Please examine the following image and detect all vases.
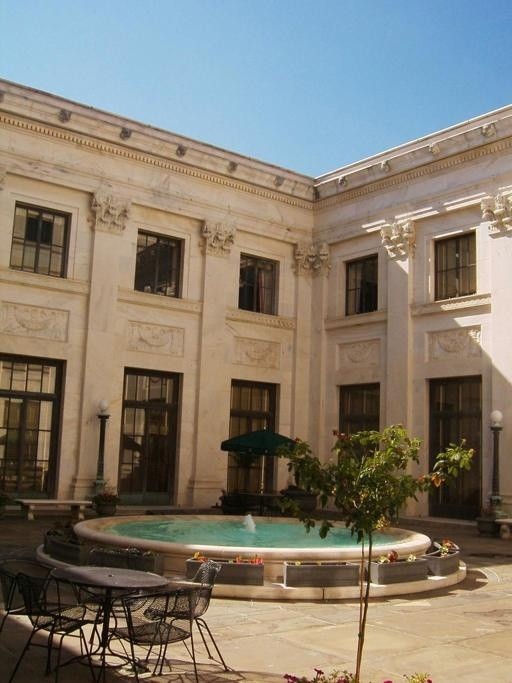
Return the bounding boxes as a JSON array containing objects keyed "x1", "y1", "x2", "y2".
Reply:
[
  {"x1": 280, "y1": 488, "x2": 320, "y2": 514},
  {"x1": 97, "y1": 503, "x2": 116, "y2": 516},
  {"x1": 475, "y1": 518, "x2": 499, "y2": 537}
]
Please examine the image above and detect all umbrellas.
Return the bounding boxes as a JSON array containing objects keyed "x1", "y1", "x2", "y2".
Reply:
[
  {"x1": 0, "y1": 431, "x2": 37, "y2": 459},
  {"x1": 220, "y1": 426, "x2": 296, "y2": 494}
]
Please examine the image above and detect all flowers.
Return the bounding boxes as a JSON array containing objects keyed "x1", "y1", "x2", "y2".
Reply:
[
  {"x1": 90, "y1": 490, "x2": 121, "y2": 504},
  {"x1": 194, "y1": 552, "x2": 208, "y2": 560},
  {"x1": 295, "y1": 560, "x2": 302, "y2": 567},
  {"x1": 378, "y1": 550, "x2": 417, "y2": 563},
  {"x1": 288, "y1": 437, "x2": 321, "y2": 492},
  {"x1": 481, "y1": 505, "x2": 496, "y2": 520},
  {"x1": 233, "y1": 553, "x2": 263, "y2": 564},
  {"x1": 441, "y1": 538, "x2": 452, "y2": 556},
  {"x1": 283, "y1": 668, "x2": 433, "y2": 683},
  {"x1": 273, "y1": 420, "x2": 476, "y2": 536}
]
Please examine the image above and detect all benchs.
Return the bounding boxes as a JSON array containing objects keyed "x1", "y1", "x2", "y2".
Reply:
[{"x1": 15, "y1": 498, "x2": 92, "y2": 520}]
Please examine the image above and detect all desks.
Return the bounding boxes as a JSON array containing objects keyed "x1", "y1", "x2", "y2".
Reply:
[{"x1": 50, "y1": 566, "x2": 170, "y2": 682}]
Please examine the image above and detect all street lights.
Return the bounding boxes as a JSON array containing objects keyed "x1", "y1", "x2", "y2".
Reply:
[
  {"x1": 86, "y1": 396, "x2": 113, "y2": 506},
  {"x1": 475, "y1": 407, "x2": 512, "y2": 541}
]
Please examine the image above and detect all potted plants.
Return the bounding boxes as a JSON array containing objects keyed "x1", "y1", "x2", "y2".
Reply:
[{"x1": 44, "y1": 521, "x2": 165, "y2": 573}]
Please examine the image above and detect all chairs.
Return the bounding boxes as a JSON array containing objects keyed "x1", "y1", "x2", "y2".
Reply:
[
  {"x1": 17, "y1": 571, "x2": 96, "y2": 683},
  {"x1": 144, "y1": 559, "x2": 226, "y2": 676},
  {"x1": 109, "y1": 589, "x2": 197, "y2": 683},
  {"x1": 0, "y1": 558, "x2": 60, "y2": 674}
]
